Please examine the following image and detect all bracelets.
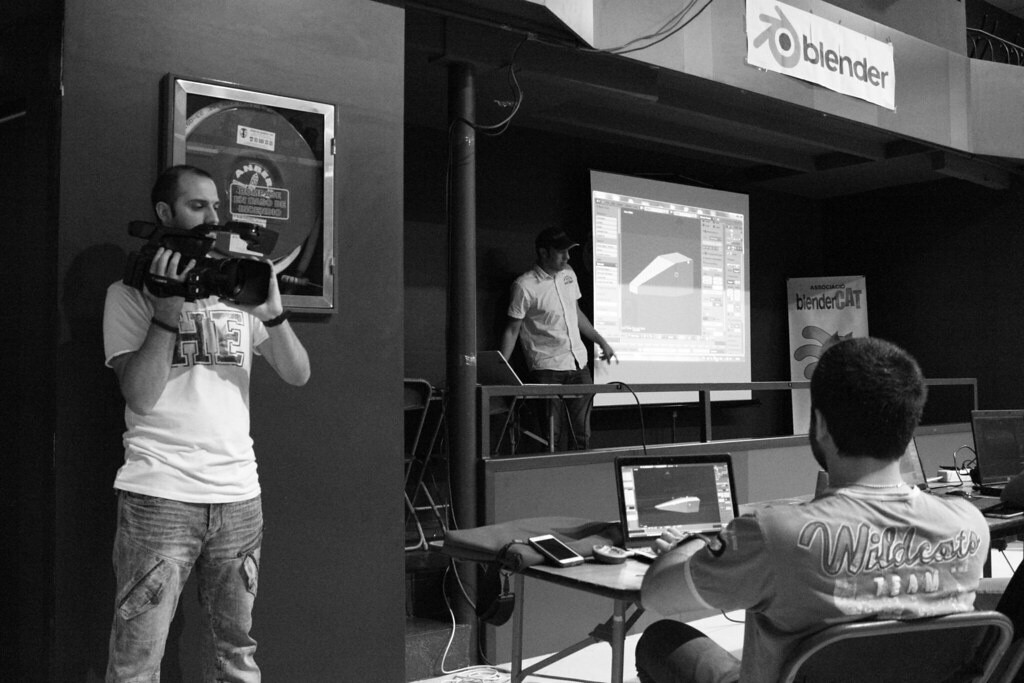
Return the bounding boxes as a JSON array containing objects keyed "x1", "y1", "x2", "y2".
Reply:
[
  {"x1": 151, "y1": 316, "x2": 179, "y2": 333},
  {"x1": 676, "y1": 533, "x2": 709, "y2": 546},
  {"x1": 263, "y1": 309, "x2": 293, "y2": 328}
]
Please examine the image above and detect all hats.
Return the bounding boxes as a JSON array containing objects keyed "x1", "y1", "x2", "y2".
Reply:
[{"x1": 535, "y1": 227, "x2": 580, "y2": 253}]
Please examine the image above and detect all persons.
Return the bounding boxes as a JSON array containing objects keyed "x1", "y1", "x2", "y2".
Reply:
[
  {"x1": 497, "y1": 228, "x2": 618, "y2": 453},
  {"x1": 103, "y1": 165, "x2": 310, "y2": 683},
  {"x1": 635, "y1": 337, "x2": 1024, "y2": 683}
]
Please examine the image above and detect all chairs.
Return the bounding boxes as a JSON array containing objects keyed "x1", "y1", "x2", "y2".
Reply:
[
  {"x1": 782, "y1": 556, "x2": 1024, "y2": 683},
  {"x1": 401, "y1": 376, "x2": 459, "y2": 554}
]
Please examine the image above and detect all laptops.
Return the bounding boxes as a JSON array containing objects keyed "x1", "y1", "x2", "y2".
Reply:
[
  {"x1": 475, "y1": 351, "x2": 562, "y2": 386},
  {"x1": 972, "y1": 408, "x2": 1024, "y2": 495},
  {"x1": 613, "y1": 454, "x2": 740, "y2": 562}
]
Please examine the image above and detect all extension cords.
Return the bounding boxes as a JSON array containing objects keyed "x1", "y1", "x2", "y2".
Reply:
[{"x1": 937, "y1": 467, "x2": 972, "y2": 483}]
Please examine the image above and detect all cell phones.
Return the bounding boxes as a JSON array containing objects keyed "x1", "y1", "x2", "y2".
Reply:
[
  {"x1": 527, "y1": 533, "x2": 585, "y2": 567},
  {"x1": 983, "y1": 507, "x2": 1024, "y2": 519}
]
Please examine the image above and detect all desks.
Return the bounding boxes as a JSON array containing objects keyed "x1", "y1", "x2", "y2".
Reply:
[
  {"x1": 429, "y1": 487, "x2": 1023, "y2": 683},
  {"x1": 497, "y1": 391, "x2": 586, "y2": 458}
]
